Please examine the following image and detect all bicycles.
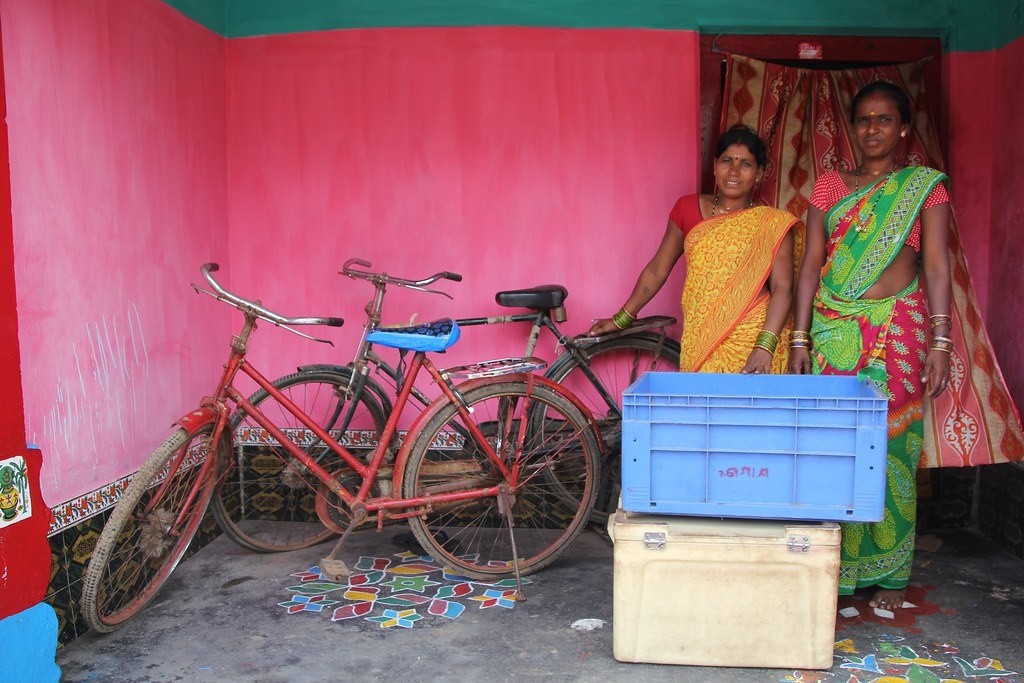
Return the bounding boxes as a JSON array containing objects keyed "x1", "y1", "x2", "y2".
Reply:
[
  {"x1": 204, "y1": 256, "x2": 681, "y2": 553},
  {"x1": 79, "y1": 263, "x2": 607, "y2": 635}
]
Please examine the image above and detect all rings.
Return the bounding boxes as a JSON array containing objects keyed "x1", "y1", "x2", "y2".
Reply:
[{"x1": 941, "y1": 380, "x2": 948, "y2": 383}]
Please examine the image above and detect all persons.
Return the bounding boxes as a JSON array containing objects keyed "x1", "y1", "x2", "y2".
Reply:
[
  {"x1": 587, "y1": 125, "x2": 806, "y2": 375},
  {"x1": 790, "y1": 84, "x2": 1024, "y2": 610}
]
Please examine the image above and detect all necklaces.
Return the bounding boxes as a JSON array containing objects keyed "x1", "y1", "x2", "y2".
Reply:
[
  {"x1": 712, "y1": 191, "x2": 753, "y2": 216},
  {"x1": 856, "y1": 164, "x2": 898, "y2": 232}
]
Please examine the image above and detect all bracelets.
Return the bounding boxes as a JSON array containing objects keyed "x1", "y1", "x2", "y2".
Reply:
[
  {"x1": 612, "y1": 307, "x2": 636, "y2": 330},
  {"x1": 929, "y1": 337, "x2": 953, "y2": 354},
  {"x1": 753, "y1": 330, "x2": 778, "y2": 358},
  {"x1": 929, "y1": 315, "x2": 953, "y2": 333},
  {"x1": 788, "y1": 330, "x2": 811, "y2": 348}
]
